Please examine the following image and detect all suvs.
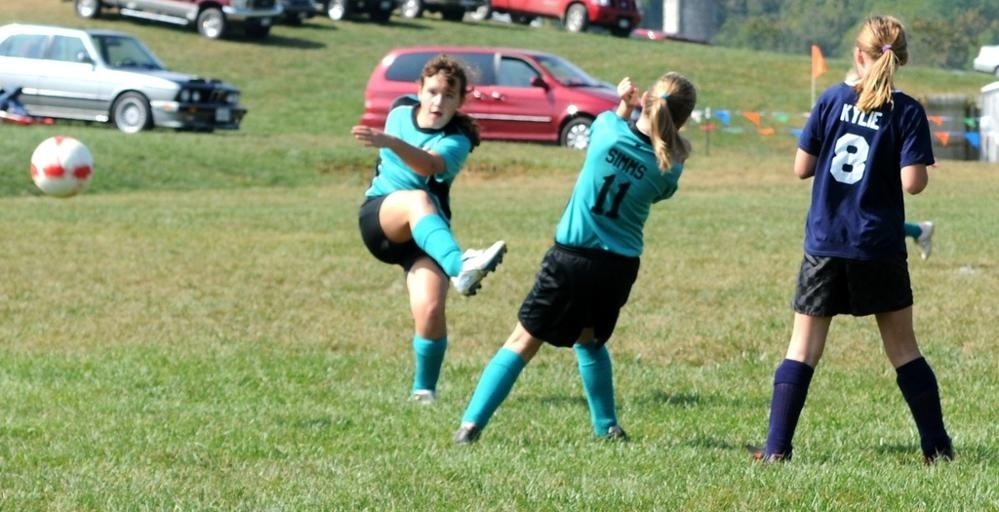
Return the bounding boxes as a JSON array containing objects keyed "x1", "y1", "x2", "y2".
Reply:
[
  {"x1": 360, "y1": 44, "x2": 644, "y2": 149},
  {"x1": 0, "y1": 23, "x2": 246, "y2": 134}
]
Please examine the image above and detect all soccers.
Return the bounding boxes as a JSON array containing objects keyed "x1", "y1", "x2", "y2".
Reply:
[{"x1": 29, "y1": 136, "x2": 92, "y2": 197}]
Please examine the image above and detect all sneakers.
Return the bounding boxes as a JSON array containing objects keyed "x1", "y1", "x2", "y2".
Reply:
[
  {"x1": 410, "y1": 389, "x2": 435, "y2": 402},
  {"x1": 915, "y1": 221, "x2": 935, "y2": 260},
  {"x1": 450, "y1": 240, "x2": 506, "y2": 296},
  {"x1": 608, "y1": 423, "x2": 624, "y2": 438},
  {"x1": 749, "y1": 445, "x2": 793, "y2": 462},
  {"x1": 453, "y1": 423, "x2": 480, "y2": 444}
]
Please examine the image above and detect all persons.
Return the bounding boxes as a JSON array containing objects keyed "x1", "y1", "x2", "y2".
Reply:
[
  {"x1": 350, "y1": 54, "x2": 507, "y2": 404},
  {"x1": 454, "y1": 70, "x2": 696, "y2": 445},
  {"x1": 905, "y1": 221, "x2": 935, "y2": 262},
  {"x1": 753, "y1": 15, "x2": 953, "y2": 465}
]
